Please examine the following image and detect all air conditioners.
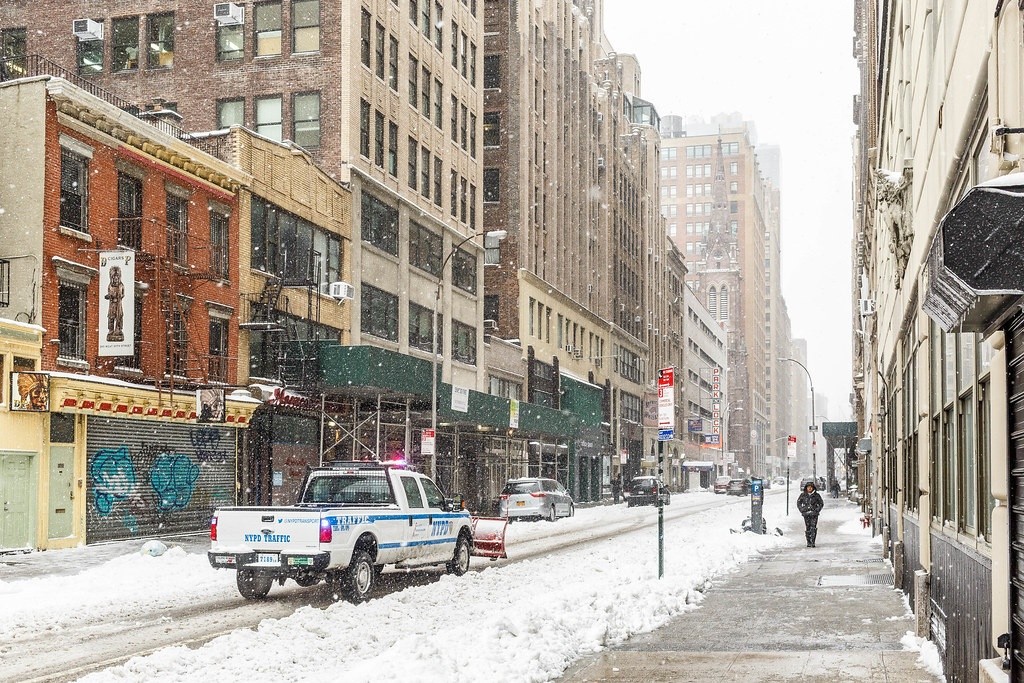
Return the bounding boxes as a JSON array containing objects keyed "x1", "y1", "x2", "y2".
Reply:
[
  {"x1": 595, "y1": 357, "x2": 600, "y2": 366},
  {"x1": 655, "y1": 329, "x2": 659, "y2": 335},
  {"x1": 599, "y1": 157, "x2": 605, "y2": 169},
  {"x1": 857, "y1": 298, "x2": 875, "y2": 317},
  {"x1": 588, "y1": 285, "x2": 592, "y2": 292},
  {"x1": 620, "y1": 303, "x2": 625, "y2": 312},
  {"x1": 856, "y1": 202, "x2": 863, "y2": 212},
  {"x1": 635, "y1": 316, "x2": 641, "y2": 322},
  {"x1": 648, "y1": 324, "x2": 652, "y2": 330},
  {"x1": 213, "y1": 2, "x2": 244, "y2": 27},
  {"x1": 483, "y1": 319, "x2": 497, "y2": 329},
  {"x1": 663, "y1": 335, "x2": 667, "y2": 341},
  {"x1": 575, "y1": 349, "x2": 582, "y2": 357},
  {"x1": 598, "y1": 111, "x2": 604, "y2": 121},
  {"x1": 853, "y1": 232, "x2": 864, "y2": 286},
  {"x1": 329, "y1": 282, "x2": 354, "y2": 306},
  {"x1": 566, "y1": 345, "x2": 573, "y2": 353},
  {"x1": 72, "y1": 18, "x2": 104, "y2": 42}
]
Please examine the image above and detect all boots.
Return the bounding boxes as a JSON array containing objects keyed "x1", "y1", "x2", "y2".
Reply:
[
  {"x1": 805, "y1": 531, "x2": 811, "y2": 547},
  {"x1": 811, "y1": 528, "x2": 817, "y2": 547}
]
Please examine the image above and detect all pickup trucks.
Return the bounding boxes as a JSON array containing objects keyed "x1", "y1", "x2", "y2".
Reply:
[{"x1": 207, "y1": 459, "x2": 510, "y2": 606}]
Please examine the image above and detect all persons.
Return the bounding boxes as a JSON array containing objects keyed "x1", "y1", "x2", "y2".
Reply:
[
  {"x1": 797, "y1": 482, "x2": 824, "y2": 548},
  {"x1": 610, "y1": 474, "x2": 622, "y2": 504},
  {"x1": 830, "y1": 480, "x2": 840, "y2": 499},
  {"x1": 617, "y1": 473, "x2": 627, "y2": 501}
]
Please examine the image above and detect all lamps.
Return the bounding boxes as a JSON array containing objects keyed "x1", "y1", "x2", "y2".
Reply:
[{"x1": 648, "y1": 248, "x2": 653, "y2": 254}]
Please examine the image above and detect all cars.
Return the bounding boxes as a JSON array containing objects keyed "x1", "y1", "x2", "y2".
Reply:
[
  {"x1": 628, "y1": 476, "x2": 671, "y2": 507},
  {"x1": 497, "y1": 477, "x2": 575, "y2": 521},
  {"x1": 761, "y1": 479, "x2": 771, "y2": 489},
  {"x1": 726, "y1": 479, "x2": 752, "y2": 496},
  {"x1": 800, "y1": 475, "x2": 827, "y2": 491},
  {"x1": 773, "y1": 476, "x2": 792, "y2": 485}
]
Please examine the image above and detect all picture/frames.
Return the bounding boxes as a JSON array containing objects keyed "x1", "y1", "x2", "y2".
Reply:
[{"x1": 9, "y1": 371, "x2": 50, "y2": 412}]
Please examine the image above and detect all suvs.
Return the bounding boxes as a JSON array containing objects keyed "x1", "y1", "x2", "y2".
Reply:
[{"x1": 714, "y1": 476, "x2": 732, "y2": 494}]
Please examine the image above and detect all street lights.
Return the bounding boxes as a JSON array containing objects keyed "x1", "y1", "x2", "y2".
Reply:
[
  {"x1": 777, "y1": 357, "x2": 816, "y2": 487},
  {"x1": 420, "y1": 230, "x2": 507, "y2": 499}
]
